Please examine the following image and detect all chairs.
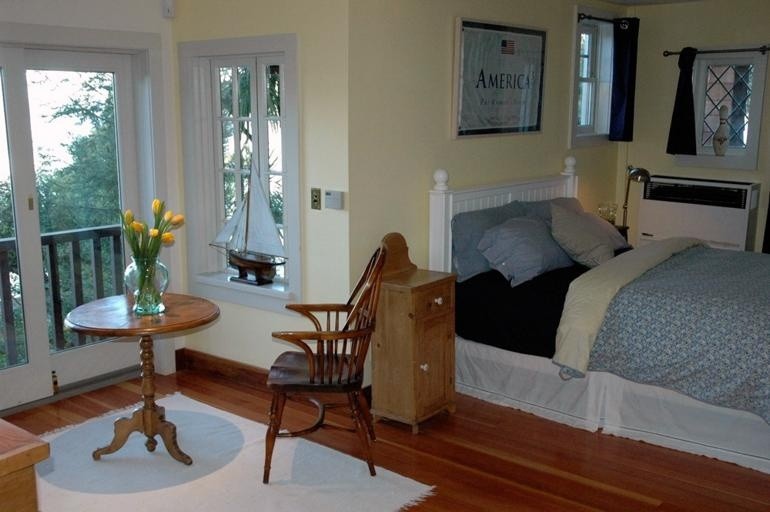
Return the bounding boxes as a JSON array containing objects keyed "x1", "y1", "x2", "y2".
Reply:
[{"x1": 262, "y1": 245, "x2": 387, "y2": 484}]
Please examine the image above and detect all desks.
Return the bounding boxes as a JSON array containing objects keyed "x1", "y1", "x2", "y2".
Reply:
[{"x1": 63, "y1": 293, "x2": 221, "y2": 466}]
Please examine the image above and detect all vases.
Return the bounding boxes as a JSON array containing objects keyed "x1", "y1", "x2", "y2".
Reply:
[{"x1": 123, "y1": 256, "x2": 170, "y2": 315}]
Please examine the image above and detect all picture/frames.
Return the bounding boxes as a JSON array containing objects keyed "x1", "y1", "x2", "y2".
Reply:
[{"x1": 451, "y1": 16, "x2": 547, "y2": 140}]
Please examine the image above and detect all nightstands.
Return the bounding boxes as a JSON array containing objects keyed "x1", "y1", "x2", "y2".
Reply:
[
  {"x1": 368, "y1": 232, "x2": 457, "y2": 435},
  {"x1": 615, "y1": 225, "x2": 629, "y2": 242}
]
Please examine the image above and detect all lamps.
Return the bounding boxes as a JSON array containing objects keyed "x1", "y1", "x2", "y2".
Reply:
[{"x1": 622, "y1": 165, "x2": 651, "y2": 227}]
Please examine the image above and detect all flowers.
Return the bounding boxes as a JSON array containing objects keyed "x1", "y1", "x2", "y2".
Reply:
[{"x1": 118, "y1": 198, "x2": 185, "y2": 307}]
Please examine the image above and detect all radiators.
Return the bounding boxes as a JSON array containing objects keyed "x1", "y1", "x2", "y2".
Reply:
[{"x1": 638, "y1": 174, "x2": 762, "y2": 251}]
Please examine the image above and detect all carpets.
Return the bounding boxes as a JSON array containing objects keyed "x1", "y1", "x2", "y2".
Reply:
[{"x1": 35, "y1": 390, "x2": 438, "y2": 511}]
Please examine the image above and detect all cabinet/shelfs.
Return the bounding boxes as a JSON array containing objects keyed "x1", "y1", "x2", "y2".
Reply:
[{"x1": 0, "y1": 418, "x2": 50, "y2": 512}]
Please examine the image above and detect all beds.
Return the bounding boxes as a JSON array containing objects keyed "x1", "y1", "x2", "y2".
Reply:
[{"x1": 429, "y1": 155, "x2": 770, "y2": 476}]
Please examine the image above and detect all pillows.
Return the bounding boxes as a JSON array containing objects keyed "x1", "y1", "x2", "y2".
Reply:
[{"x1": 451, "y1": 198, "x2": 634, "y2": 290}]
